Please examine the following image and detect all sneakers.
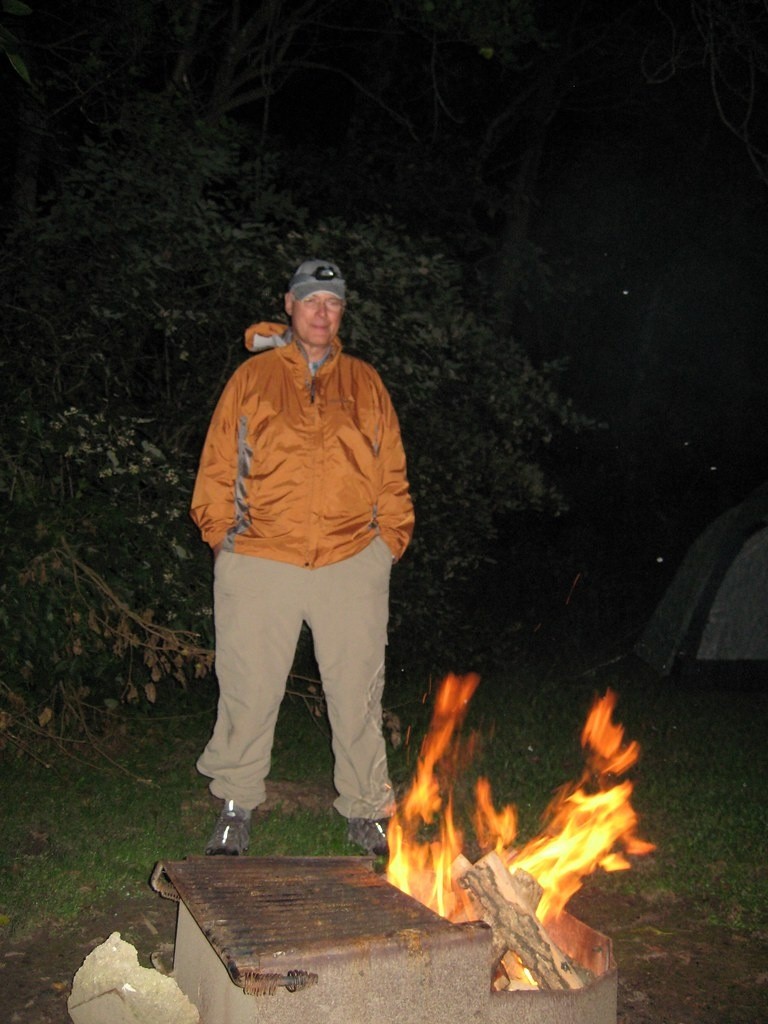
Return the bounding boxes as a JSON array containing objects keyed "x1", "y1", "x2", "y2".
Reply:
[
  {"x1": 347, "y1": 817, "x2": 390, "y2": 858},
  {"x1": 204, "y1": 801, "x2": 250, "y2": 854}
]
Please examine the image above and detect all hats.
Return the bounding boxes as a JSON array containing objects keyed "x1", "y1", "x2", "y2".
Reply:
[{"x1": 289, "y1": 257, "x2": 345, "y2": 303}]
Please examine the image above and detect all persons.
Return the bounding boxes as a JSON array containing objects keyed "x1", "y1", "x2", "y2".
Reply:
[{"x1": 189, "y1": 263, "x2": 416, "y2": 851}]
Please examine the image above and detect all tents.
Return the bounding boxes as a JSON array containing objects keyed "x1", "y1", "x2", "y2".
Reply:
[{"x1": 633, "y1": 486, "x2": 768, "y2": 690}]
